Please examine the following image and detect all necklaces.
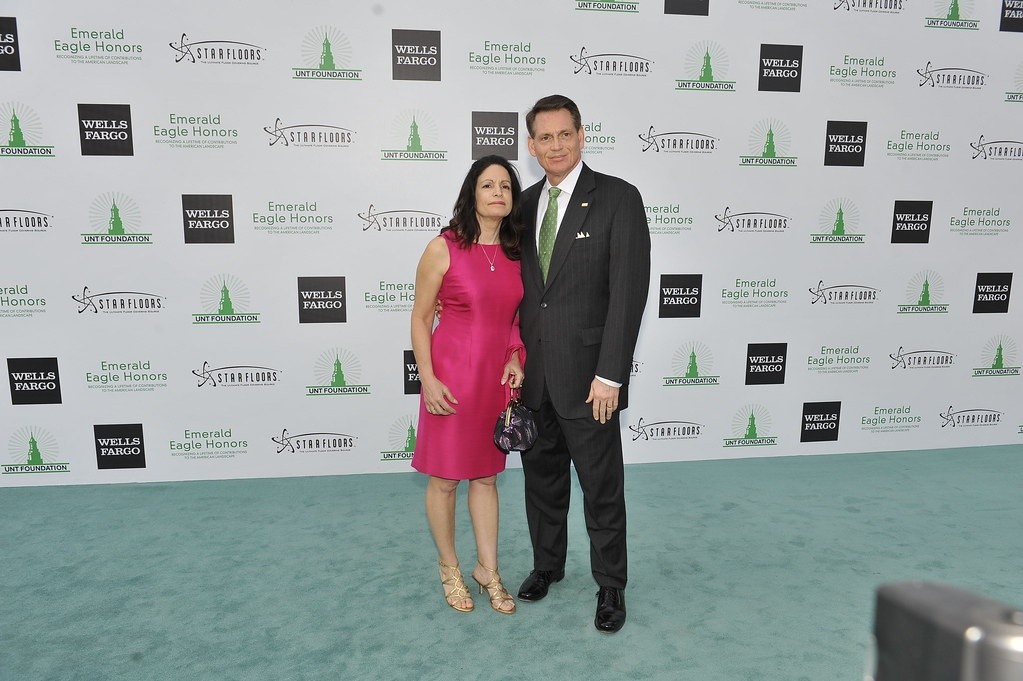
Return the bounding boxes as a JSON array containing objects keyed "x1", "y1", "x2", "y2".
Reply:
[{"x1": 472, "y1": 233, "x2": 506, "y2": 272}]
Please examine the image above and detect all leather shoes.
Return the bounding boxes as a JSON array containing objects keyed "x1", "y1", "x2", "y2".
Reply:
[
  {"x1": 595, "y1": 587, "x2": 628, "y2": 634},
  {"x1": 516, "y1": 567, "x2": 566, "y2": 602}
]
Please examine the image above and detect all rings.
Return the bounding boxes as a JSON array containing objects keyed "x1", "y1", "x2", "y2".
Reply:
[{"x1": 606, "y1": 405, "x2": 612, "y2": 409}]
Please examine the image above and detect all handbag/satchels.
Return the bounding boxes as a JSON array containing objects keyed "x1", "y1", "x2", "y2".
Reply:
[{"x1": 493, "y1": 401, "x2": 537, "y2": 451}]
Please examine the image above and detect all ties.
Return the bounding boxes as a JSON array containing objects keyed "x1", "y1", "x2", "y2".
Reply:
[{"x1": 537, "y1": 187, "x2": 561, "y2": 288}]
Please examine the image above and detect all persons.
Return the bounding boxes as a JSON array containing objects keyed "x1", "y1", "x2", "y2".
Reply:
[
  {"x1": 410, "y1": 156, "x2": 527, "y2": 615},
  {"x1": 501, "y1": 95, "x2": 651, "y2": 636}
]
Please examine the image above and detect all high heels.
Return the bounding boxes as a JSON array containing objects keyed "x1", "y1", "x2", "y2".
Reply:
[
  {"x1": 469, "y1": 560, "x2": 517, "y2": 616},
  {"x1": 436, "y1": 561, "x2": 476, "y2": 613}
]
